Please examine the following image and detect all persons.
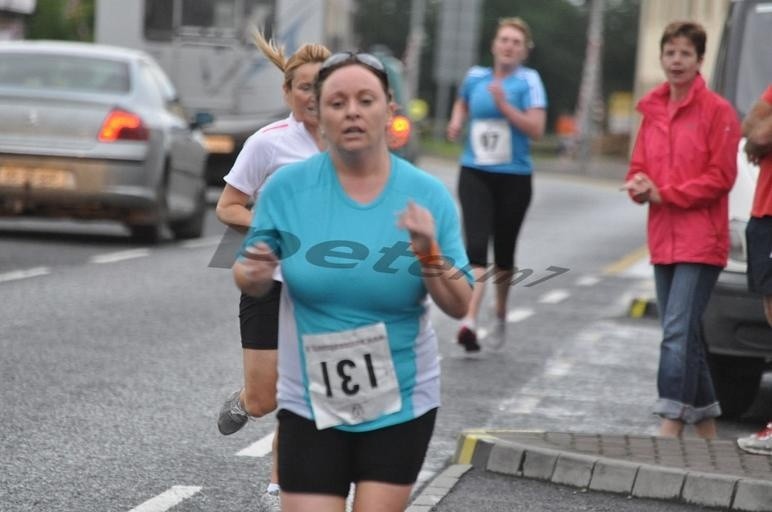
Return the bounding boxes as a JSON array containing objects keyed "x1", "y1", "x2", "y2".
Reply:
[
  {"x1": 231, "y1": 50, "x2": 476, "y2": 511},
  {"x1": 442, "y1": 15, "x2": 549, "y2": 353},
  {"x1": 215, "y1": 34, "x2": 332, "y2": 494},
  {"x1": 737, "y1": 85, "x2": 772, "y2": 455},
  {"x1": 617, "y1": 19, "x2": 742, "y2": 442}
]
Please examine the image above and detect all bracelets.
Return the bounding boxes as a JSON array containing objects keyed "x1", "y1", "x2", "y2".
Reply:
[{"x1": 410, "y1": 243, "x2": 439, "y2": 266}]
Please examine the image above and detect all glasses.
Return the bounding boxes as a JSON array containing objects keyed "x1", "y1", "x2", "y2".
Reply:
[{"x1": 322, "y1": 50, "x2": 386, "y2": 76}]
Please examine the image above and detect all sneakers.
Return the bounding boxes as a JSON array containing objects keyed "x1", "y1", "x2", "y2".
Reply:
[
  {"x1": 456, "y1": 314, "x2": 507, "y2": 352},
  {"x1": 737, "y1": 421, "x2": 772, "y2": 455},
  {"x1": 217, "y1": 385, "x2": 282, "y2": 512}
]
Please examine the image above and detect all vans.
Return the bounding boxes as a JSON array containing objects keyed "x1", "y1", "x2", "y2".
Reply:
[{"x1": 701, "y1": 0, "x2": 772, "y2": 422}]
